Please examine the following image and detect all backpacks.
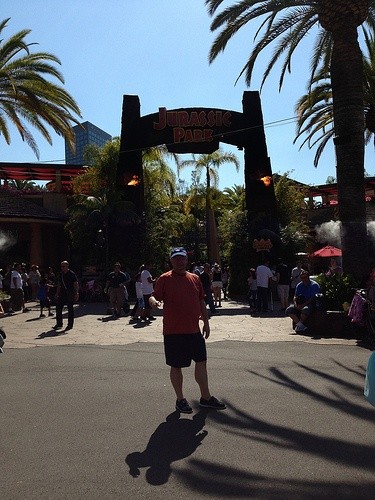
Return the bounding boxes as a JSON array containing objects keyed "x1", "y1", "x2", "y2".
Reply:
[{"x1": 37, "y1": 286, "x2": 46, "y2": 300}]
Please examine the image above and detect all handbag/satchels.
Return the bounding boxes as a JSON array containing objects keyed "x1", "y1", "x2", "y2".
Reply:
[{"x1": 71, "y1": 293, "x2": 80, "y2": 301}]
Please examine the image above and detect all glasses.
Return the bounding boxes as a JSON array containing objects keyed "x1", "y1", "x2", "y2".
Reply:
[{"x1": 301, "y1": 277, "x2": 306, "y2": 279}]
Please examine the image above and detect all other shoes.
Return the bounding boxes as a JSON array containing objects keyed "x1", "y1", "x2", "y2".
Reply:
[
  {"x1": 176, "y1": 398, "x2": 193, "y2": 413},
  {"x1": 200, "y1": 395, "x2": 226, "y2": 409}
]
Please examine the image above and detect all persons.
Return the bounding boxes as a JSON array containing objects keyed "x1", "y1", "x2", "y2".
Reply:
[
  {"x1": 0, "y1": 262, "x2": 57, "y2": 312},
  {"x1": 37, "y1": 278, "x2": 54, "y2": 317},
  {"x1": 189, "y1": 260, "x2": 229, "y2": 316},
  {"x1": 247, "y1": 258, "x2": 291, "y2": 317},
  {"x1": 324, "y1": 257, "x2": 343, "y2": 276},
  {"x1": 130, "y1": 263, "x2": 158, "y2": 328},
  {"x1": 290, "y1": 261, "x2": 304, "y2": 304},
  {"x1": 285, "y1": 270, "x2": 320, "y2": 334},
  {"x1": 103, "y1": 263, "x2": 131, "y2": 318},
  {"x1": 149, "y1": 247, "x2": 226, "y2": 413},
  {"x1": 52, "y1": 261, "x2": 79, "y2": 331},
  {"x1": 362, "y1": 265, "x2": 375, "y2": 320}
]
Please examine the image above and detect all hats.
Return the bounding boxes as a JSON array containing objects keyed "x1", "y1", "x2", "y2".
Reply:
[{"x1": 170, "y1": 247, "x2": 187, "y2": 259}]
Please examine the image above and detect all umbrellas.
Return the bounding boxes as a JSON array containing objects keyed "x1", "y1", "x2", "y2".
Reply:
[{"x1": 309, "y1": 244, "x2": 342, "y2": 259}]
